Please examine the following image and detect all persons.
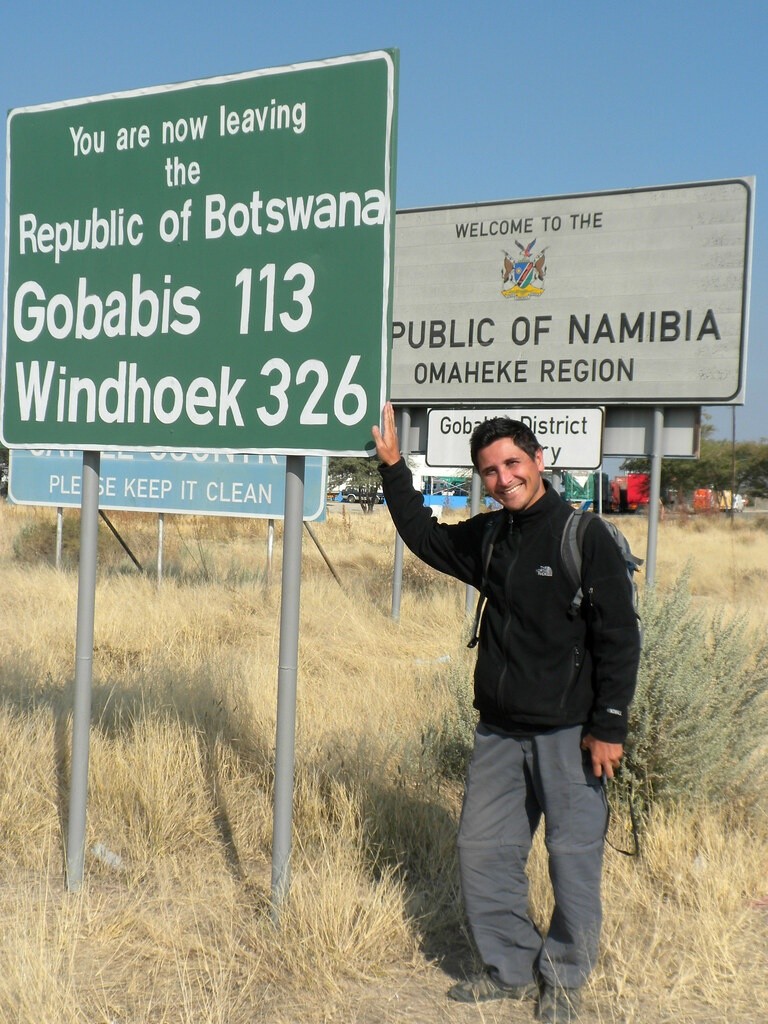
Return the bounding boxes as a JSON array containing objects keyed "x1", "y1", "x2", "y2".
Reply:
[{"x1": 369, "y1": 400, "x2": 644, "y2": 1024}]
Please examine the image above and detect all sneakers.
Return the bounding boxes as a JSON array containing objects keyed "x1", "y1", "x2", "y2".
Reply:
[
  {"x1": 533, "y1": 985, "x2": 579, "y2": 1023},
  {"x1": 448, "y1": 973, "x2": 536, "y2": 1004}
]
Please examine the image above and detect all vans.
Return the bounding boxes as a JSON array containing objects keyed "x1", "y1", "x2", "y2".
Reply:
[{"x1": 342, "y1": 485, "x2": 385, "y2": 504}]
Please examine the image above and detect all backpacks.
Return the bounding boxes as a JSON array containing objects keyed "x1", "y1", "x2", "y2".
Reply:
[{"x1": 467, "y1": 513, "x2": 642, "y2": 653}]
